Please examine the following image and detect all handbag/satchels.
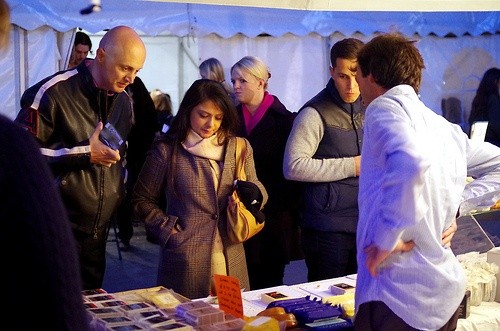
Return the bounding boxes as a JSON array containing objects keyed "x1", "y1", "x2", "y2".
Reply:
[{"x1": 227, "y1": 137, "x2": 265, "y2": 243}]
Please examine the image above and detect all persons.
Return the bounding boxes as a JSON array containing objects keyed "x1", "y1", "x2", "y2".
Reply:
[
  {"x1": 114, "y1": 76, "x2": 174, "y2": 253},
  {"x1": 284, "y1": 38, "x2": 368, "y2": 282},
  {"x1": 67, "y1": 32, "x2": 92, "y2": 71},
  {"x1": 354, "y1": 31, "x2": 500, "y2": 331},
  {"x1": 231, "y1": 56, "x2": 305, "y2": 290},
  {"x1": 0, "y1": 0, "x2": 91, "y2": 331},
  {"x1": 199, "y1": 58, "x2": 241, "y2": 107},
  {"x1": 468, "y1": 68, "x2": 500, "y2": 147},
  {"x1": 135, "y1": 79, "x2": 268, "y2": 300},
  {"x1": 13, "y1": 25, "x2": 147, "y2": 294}
]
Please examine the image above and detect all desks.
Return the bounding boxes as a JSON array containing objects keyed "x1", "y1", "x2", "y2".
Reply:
[{"x1": 190, "y1": 272, "x2": 358, "y2": 331}]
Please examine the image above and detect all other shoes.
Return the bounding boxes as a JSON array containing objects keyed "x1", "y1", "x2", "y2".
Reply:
[
  {"x1": 146, "y1": 235, "x2": 156, "y2": 243},
  {"x1": 119, "y1": 240, "x2": 129, "y2": 250}
]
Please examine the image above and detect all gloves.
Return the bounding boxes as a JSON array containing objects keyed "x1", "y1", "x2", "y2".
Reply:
[{"x1": 237, "y1": 180, "x2": 266, "y2": 224}]
